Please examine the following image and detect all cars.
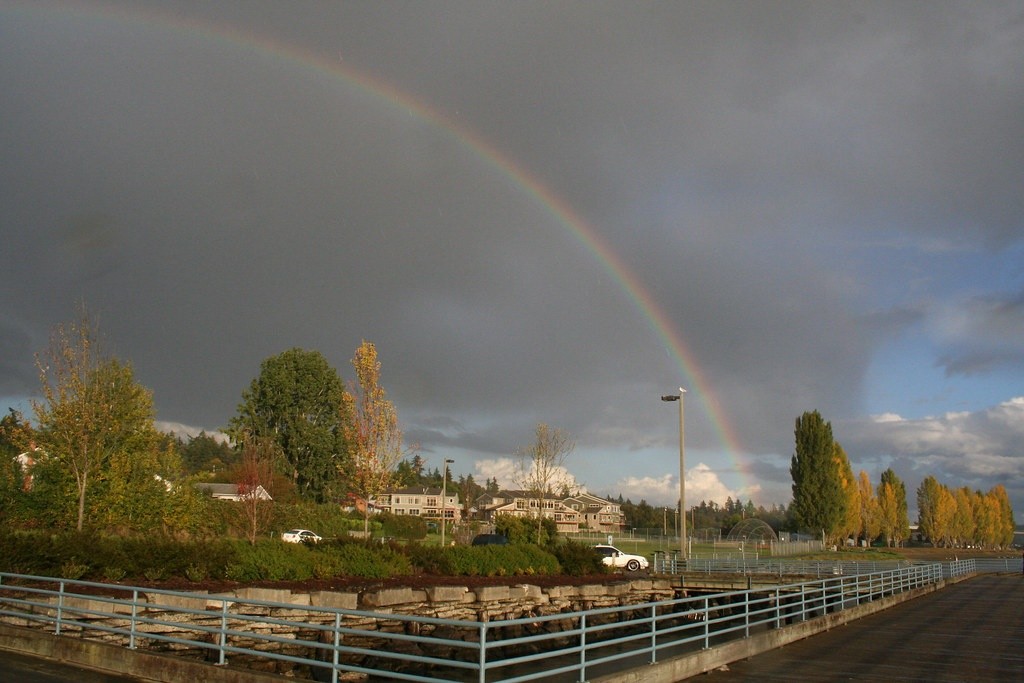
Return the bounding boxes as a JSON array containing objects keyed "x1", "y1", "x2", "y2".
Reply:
[
  {"x1": 593, "y1": 545, "x2": 650, "y2": 571},
  {"x1": 281, "y1": 528, "x2": 322, "y2": 545}
]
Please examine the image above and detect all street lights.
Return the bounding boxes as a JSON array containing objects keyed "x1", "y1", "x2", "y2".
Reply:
[
  {"x1": 660, "y1": 386, "x2": 687, "y2": 560},
  {"x1": 441, "y1": 458, "x2": 455, "y2": 548}
]
